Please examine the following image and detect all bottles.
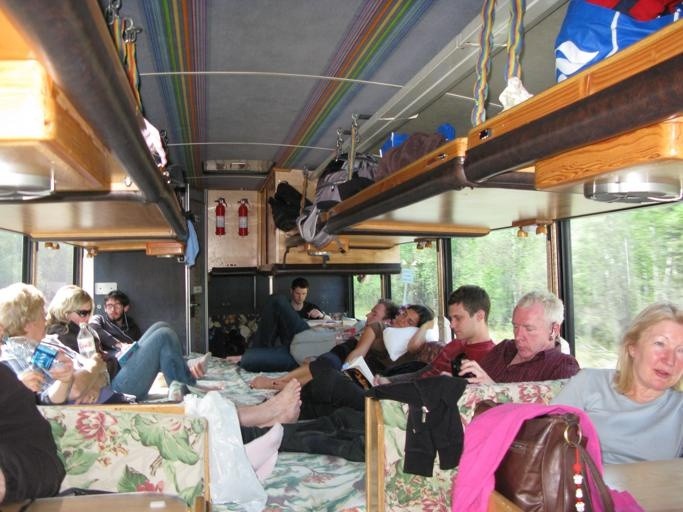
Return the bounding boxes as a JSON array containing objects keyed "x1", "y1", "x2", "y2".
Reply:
[
  {"x1": 2, "y1": 335, "x2": 64, "y2": 371},
  {"x1": 77, "y1": 322, "x2": 96, "y2": 358}
]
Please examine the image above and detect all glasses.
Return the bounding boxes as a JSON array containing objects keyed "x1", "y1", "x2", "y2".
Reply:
[
  {"x1": 74, "y1": 309, "x2": 91, "y2": 317},
  {"x1": 105, "y1": 303, "x2": 121, "y2": 309}
]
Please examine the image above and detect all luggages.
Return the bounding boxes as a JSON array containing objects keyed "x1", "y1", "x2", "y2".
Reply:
[{"x1": 97, "y1": 0, "x2": 185, "y2": 188}]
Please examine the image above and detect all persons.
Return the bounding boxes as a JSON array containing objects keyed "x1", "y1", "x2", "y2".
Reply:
[
  {"x1": 240, "y1": 288, "x2": 581, "y2": 461},
  {"x1": 1, "y1": 282, "x2": 303, "y2": 430},
  {"x1": 221, "y1": 278, "x2": 435, "y2": 373},
  {"x1": 550, "y1": 303, "x2": 683, "y2": 461},
  {"x1": 0, "y1": 325, "x2": 284, "y2": 485},
  {"x1": 299, "y1": 285, "x2": 496, "y2": 420},
  {"x1": 0, "y1": 363, "x2": 66, "y2": 505}
]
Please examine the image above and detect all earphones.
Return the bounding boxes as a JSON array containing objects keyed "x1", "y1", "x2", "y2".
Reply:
[{"x1": 124, "y1": 307, "x2": 127, "y2": 311}]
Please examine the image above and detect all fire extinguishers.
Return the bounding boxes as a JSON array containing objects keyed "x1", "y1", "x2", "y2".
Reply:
[
  {"x1": 215, "y1": 197, "x2": 227, "y2": 235},
  {"x1": 237, "y1": 198, "x2": 249, "y2": 236}
]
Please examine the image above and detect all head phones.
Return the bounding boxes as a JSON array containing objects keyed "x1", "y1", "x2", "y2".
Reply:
[{"x1": 105, "y1": 307, "x2": 106, "y2": 312}]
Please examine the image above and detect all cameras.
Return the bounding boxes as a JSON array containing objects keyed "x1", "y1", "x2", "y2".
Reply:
[{"x1": 451, "y1": 352, "x2": 472, "y2": 378}]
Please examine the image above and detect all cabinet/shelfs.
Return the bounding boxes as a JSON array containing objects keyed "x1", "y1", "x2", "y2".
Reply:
[{"x1": 263, "y1": 166, "x2": 404, "y2": 274}]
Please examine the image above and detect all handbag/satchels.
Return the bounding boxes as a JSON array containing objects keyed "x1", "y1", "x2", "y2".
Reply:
[
  {"x1": 554, "y1": 0, "x2": 683, "y2": 84},
  {"x1": 268, "y1": 123, "x2": 455, "y2": 249},
  {"x1": 472, "y1": 400, "x2": 614, "y2": 512}
]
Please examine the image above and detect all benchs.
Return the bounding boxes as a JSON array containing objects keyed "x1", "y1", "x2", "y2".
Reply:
[
  {"x1": 363, "y1": 378, "x2": 569, "y2": 512},
  {"x1": 37, "y1": 403, "x2": 210, "y2": 512}
]
